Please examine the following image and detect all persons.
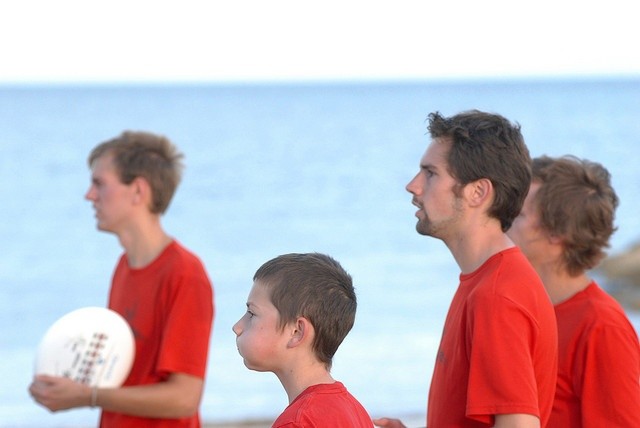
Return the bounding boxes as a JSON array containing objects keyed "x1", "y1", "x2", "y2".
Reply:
[
  {"x1": 232, "y1": 253, "x2": 373, "y2": 428},
  {"x1": 29, "y1": 130, "x2": 214, "y2": 428},
  {"x1": 374, "y1": 109, "x2": 558, "y2": 428},
  {"x1": 504, "y1": 155, "x2": 640, "y2": 428}
]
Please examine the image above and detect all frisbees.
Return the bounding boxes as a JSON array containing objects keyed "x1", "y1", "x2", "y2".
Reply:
[{"x1": 37, "y1": 307, "x2": 135, "y2": 388}]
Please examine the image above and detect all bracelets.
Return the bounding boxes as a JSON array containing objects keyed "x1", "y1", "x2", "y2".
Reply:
[{"x1": 91, "y1": 386, "x2": 96, "y2": 408}]
